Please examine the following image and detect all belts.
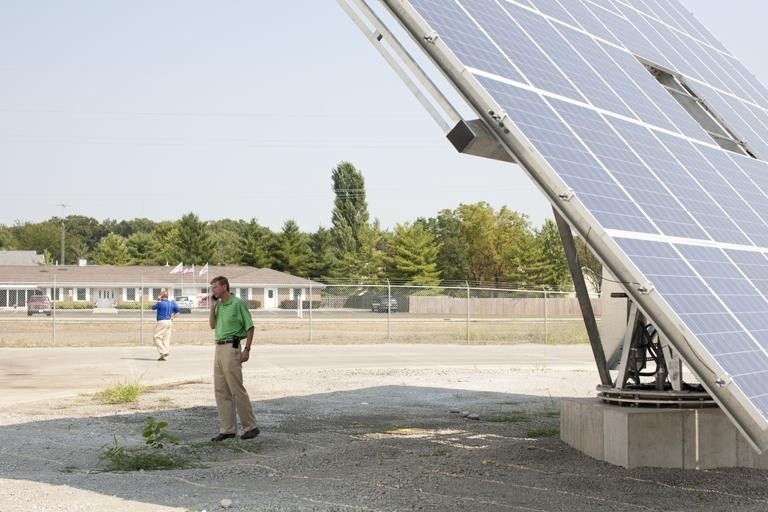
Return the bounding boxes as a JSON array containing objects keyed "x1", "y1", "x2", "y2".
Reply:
[{"x1": 216, "y1": 340, "x2": 233, "y2": 345}]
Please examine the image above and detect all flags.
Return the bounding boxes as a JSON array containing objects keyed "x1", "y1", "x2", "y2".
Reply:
[
  {"x1": 183, "y1": 264, "x2": 193, "y2": 274},
  {"x1": 169, "y1": 262, "x2": 184, "y2": 274},
  {"x1": 199, "y1": 262, "x2": 208, "y2": 277}
]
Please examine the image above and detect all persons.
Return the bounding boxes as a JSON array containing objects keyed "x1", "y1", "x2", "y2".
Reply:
[
  {"x1": 150, "y1": 290, "x2": 181, "y2": 361},
  {"x1": 209, "y1": 276, "x2": 262, "y2": 442}
]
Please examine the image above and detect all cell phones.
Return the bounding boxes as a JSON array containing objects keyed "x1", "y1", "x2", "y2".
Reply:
[{"x1": 212, "y1": 295, "x2": 217, "y2": 301}]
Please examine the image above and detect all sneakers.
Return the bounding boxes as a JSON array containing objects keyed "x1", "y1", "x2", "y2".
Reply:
[{"x1": 158, "y1": 354, "x2": 169, "y2": 361}]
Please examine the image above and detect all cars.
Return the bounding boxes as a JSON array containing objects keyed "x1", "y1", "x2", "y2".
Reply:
[
  {"x1": 26, "y1": 295, "x2": 52, "y2": 316},
  {"x1": 371, "y1": 295, "x2": 398, "y2": 312}
]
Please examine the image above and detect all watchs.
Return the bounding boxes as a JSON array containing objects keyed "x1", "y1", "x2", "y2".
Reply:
[{"x1": 244, "y1": 346, "x2": 251, "y2": 352}]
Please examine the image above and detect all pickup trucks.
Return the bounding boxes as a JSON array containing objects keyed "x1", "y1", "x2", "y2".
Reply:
[{"x1": 173, "y1": 296, "x2": 194, "y2": 315}]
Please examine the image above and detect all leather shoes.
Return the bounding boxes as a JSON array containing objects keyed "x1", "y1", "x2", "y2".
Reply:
[
  {"x1": 240, "y1": 427, "x2": 260, "y2": 439},
  {"x1": 211, "y1": 433, "x2": 235, "y2": 441}
]
